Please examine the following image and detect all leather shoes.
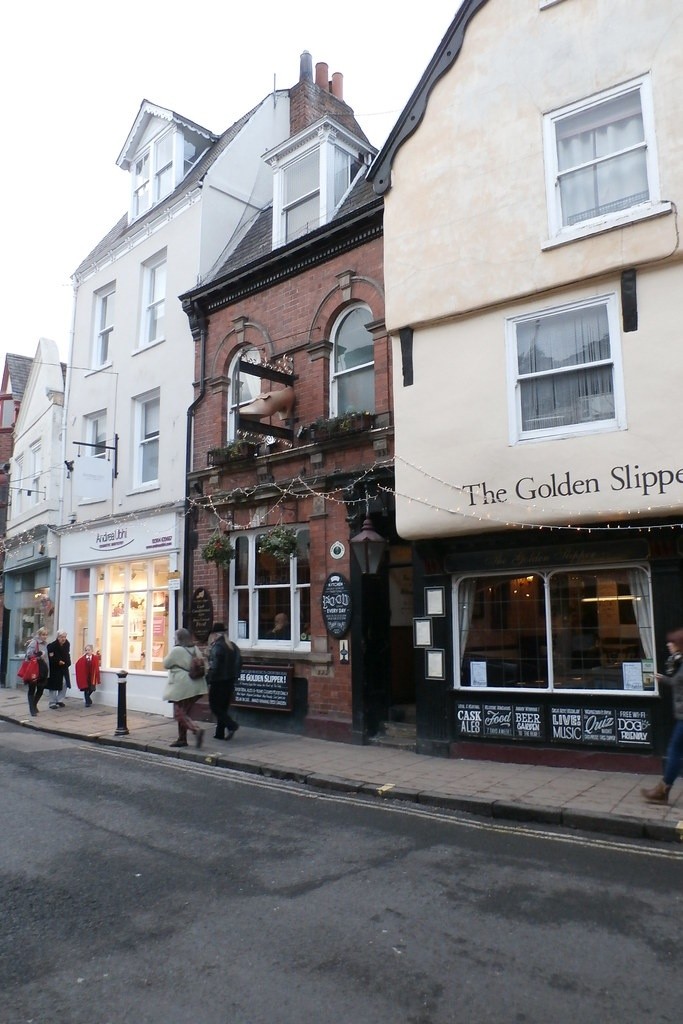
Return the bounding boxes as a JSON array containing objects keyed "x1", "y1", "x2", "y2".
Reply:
[
  {"x1": 85, "y1": 700, "x2": 92, "y2": 707},
  {"x1": 213, "y1": 724, "x2": 238, "y2": 740},
  {"x1": 169, "y1": 726, "x2": 205, "y2": 749},
  {"x1": 49, "y1": 703, "x2": 65, "y2": 709}
]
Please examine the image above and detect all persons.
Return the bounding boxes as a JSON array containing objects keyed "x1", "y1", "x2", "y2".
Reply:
[
  {"x1": 46, "y1": 630, "x2": 71, "y2": 710},
  {"x1": 23, "y1": 627, "x2": 50, "y2": 716},
  {"x1": 75, "y1": 645, "x2": 100, "y2": 707},
  {"x1": 261, "y1": 614, "x2": 290, "y2": 640},
  {"x1": 640, "y1": 631, "x2": 683, "y2": 806},
  {"x1": 161, "y1": 628, "x2": 209, "y2": 747},
  {"x1": 204, "y1": 623, "x2": 241, "y2": 741}
]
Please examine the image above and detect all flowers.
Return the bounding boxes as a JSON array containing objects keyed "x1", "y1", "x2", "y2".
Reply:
[
  {"x1": 202, "y1": 536, "x2": 236, "y2": 565},
  {"x1": 258, "y1": 526, "x2": 303, "y2": 564}
]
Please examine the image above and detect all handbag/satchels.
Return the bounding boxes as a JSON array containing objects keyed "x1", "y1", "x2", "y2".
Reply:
[{"x1": 18, "y1": 656, "x2": 48, "y2": 685}]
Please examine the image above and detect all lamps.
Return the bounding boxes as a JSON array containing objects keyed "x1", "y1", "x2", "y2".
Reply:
[{"x1": 348, "y1": 511, "x2": 415, "y2": 576}]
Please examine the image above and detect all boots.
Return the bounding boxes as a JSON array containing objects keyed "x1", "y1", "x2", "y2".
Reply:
[
  {"x1": 30, "y1": 703, "x2": 39, "y2": 716},
  {"x1": 640, "y1": 778, "x2": 672, "y2": 805}
]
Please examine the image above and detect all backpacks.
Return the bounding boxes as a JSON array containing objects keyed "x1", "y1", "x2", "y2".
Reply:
[{"x1": 188, "y1": 656, "x2": 206, "y2": 680}]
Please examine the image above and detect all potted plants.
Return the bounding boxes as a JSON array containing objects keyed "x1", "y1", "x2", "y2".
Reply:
[
  {"x1": 312, "y1": 410, "x2": 377, "y2": 442},
  {"x1": 230, "y1": 440, "x2": 254, "y2": 458}
]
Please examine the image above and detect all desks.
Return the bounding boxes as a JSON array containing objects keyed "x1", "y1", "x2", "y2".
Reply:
[{"x1": 505, "y1": 675, "x2": 599, "y2": 688}]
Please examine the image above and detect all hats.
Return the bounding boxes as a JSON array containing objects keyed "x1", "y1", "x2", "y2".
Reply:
[{"x1": 208, "y1": 622, "x2": 227, "y2": 632}]
